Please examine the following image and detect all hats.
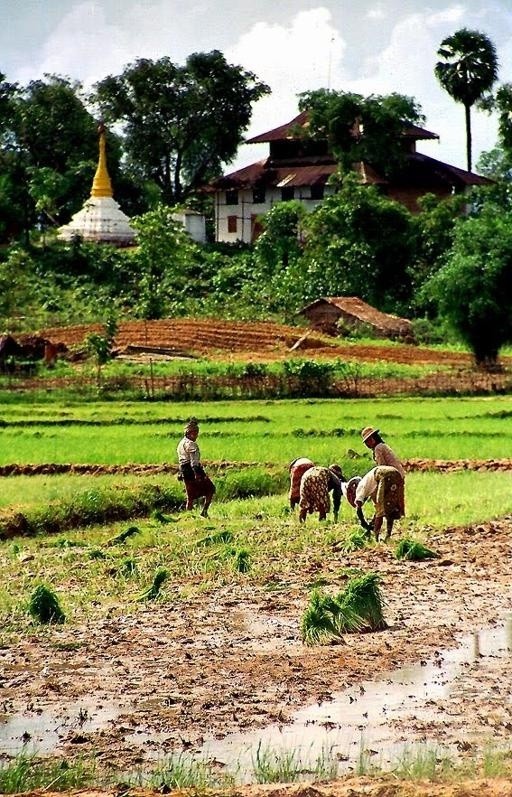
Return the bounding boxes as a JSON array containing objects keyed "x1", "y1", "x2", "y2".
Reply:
[
  {"x1": 328, "y1": 464, "x2": 342, "y2": 478},
  {"x1": 346, "y1": 476, "x2": 361, "y2": 508},
  {"x1": 361, "y1": 427, "x2": 380, "y2": 443}
]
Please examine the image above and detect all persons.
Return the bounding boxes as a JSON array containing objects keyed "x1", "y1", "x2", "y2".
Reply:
[
  {"x1": 288, "y1": 427, "x2": 405, "y2": 542},
  {"x1": 176, "y1": 421, "x2": 216, "y2": 520}
]
